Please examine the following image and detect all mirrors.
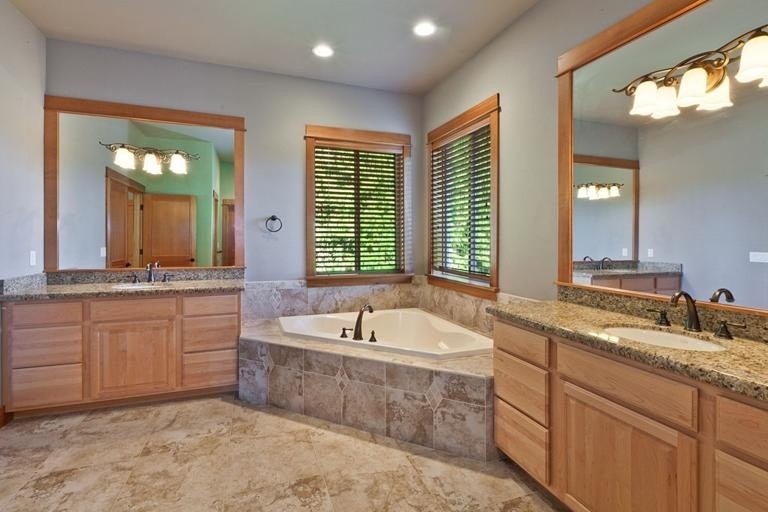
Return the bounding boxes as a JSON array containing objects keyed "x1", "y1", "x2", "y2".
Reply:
[
  {"x1": 572, "y1": 154, "x2": 640, "y2": 263},
  {"x1": 44, "y1": 94, "x2": 246, "y2": 272},
  {"x1": 555, "y1": 0, "x2": 768, "y2": 316}
]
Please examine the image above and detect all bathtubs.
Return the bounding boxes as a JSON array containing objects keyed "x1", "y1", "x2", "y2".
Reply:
[{"x1": 278, "y1": 307, "x2": 493, "y2": 361}]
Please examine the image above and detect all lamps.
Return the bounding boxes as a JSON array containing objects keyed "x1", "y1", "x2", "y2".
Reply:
[
  {"x1": 573, "y1": 181, "x2": 625, "y2": 200},
  {"x1": 612, "y1": 23, "x2": 768, "y2": 115},
  {"x1": 98, "y1": 140, "x2": 201, "y2": 176},
  {"x1": 651, "y1": 57, "x2": 768, "y2": 119}
]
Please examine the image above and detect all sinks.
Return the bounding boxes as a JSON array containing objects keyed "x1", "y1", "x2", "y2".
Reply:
[
  {"x1": 598, "y1": 321, "x2": 730, "y2": 353},
  {"x1": 111, "y1": 282, "x2": 173, "y2": 288}
]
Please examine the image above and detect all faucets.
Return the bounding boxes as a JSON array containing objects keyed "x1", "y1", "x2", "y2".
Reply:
[
  {"x1": 146, "y1": 262, "x2": 154, "y2": 283},
  {"x1": 668, "y1": 289, "x2": 702, "y2": 332},
  {"x1": 598, "y1": 256, "x2": 613, "y2": 270},
  {"x1": 583, "y1": 256, "x2": 595, "y2": 261},
  {"x1": 154, "y1": 259, "x2": 161, "y2": 268},
  {"x1": 710, "y1": 287, "x2": 736, "y2": 304},
  {"x1": 352, "y1": 303, "x2": 373, "y2": 340}
]
